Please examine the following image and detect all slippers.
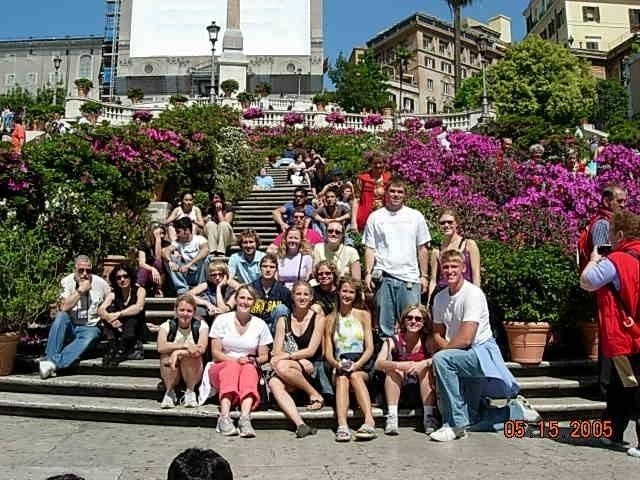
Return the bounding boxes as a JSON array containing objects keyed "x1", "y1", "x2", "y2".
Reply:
[
  {"x1": 295, "y1": 423, "x2": 317, "y2": 437},
  {"x1": 307, "y1": 398, "x2": 324, "y2": 411},
  {"x1": 355, "y1": 425, "x2": 377, "y2": 439},
  {"x1": 336, "y1": 427, "x2": 352, "y2": 441}
]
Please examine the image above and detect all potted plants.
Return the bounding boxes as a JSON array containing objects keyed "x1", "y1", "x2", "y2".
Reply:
[
  {"x1": 221, "y1": 79, "x2": 239, "y2": 97},
  {"x1": 80, "y1": 101, "x2": 102, "y2": 123},
  {"x1": 169, "y1": 94, "x2": 189, "y2": 108},
  {"x1": 45, "y1": 104, "x2": 65, "y2": 121},
  {"x1": 236, "y1": 92, "x2": 255, "y2": 109},
  {"x1": 74, "y1": 78, "x2": 93, "y2": 97},
  {"x1": 101, "y1": 211, "x2": 146, "y2": 283},
  {"x1": 255, "y1": 81, "x2": 273, "y2": 97},
  {"x1": 576, "y1": 262, "x2": 599, "y2": 360},
  {"x1": 488, "y1": 113, "x2": 524, "y2": 151},
  {"x1": 128, "y1": 149, "x2": 184, "y2": 227},
  {"x1": 27, "y1": 106, "x2": 47, "y2": 132},
  {"x1": 0, "y1": 196, "x2": 64, "y2": 377},
  {"x1": 480, "y1": 241, "x2": 580, "y2": 364},
  {"x1": 127, "y1": 87, "x2": 143, "y2": 104},
  {"x1": 313, "y1": 94, "x2": 328, "y2": 112},
  {"x1": 381, "y1": 101, "x2": 397, "y2": 115}
]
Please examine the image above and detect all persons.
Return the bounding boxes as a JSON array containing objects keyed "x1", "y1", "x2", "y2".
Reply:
[
  {"x1": 137, "y1": 221, "x2": 171, "y2": 298},
  {"x1": 248, "y1": 254, "x2": 291, "y2": 339},
  {"x1": 164, "y1": 217, "x2": 215, "y2": 298},
  {"x1": 184, "y1": 262, "x2": 238, "y2": 329},
  {"x1": 51, "y1": 111, "x2": 74, "y2": 135},
  {"x1": 308, "y1": 148, "x2": 326, "y2": 185},
  {"x1": 336, "y1": 185, "x2": 353, "y2": 210},
  {"x1": 351, "y1": 154, "x2": 391, "y2": 234},
  {"x1": 203, "y1": 188, "x2": 233, "y2": 258},
  {"x1": 274, "y1": 226, "x2": 312, "y2": 290},
  {"x1": 425, "y1": 116, "x2": 451, "y2": 152},
  {"x1": 361, "y1": 178, "x2": 432, "y2": 359},
  {"x1": 304, "y1": 151, "x2": 314, "y2": 169},
  {"x1": 287, "y1": 153, "x2": 311, "y2": 185},
  {"x1": 578, "y1": 186, "x2": 626, "y2": 367},
  {"x1": 313, "y1": 220, "x2": 361, "y2": 288},
  {"x1": 0, "y1": 104, "x2": 16, "y2": 134},
  {"x1": 312, "y1": 171, "x2": 354, "y2": 199},
  {"x1": 562, "y1": 123, "x2": 609, "y2": 176},
  {"x1": 227, "y1": 228, "x2": 268, "y2": 291},
  {"x1": 374, "y1": 303, "x2": 440, "y2": 435},
  {"x1": 311, "y1": 260, "x2": 340, "y2": 318},
  {"x1": 12, "y1": 117, "x2": 26, "y2": 155},
  {"x1": 312, "y1": 189, "x2": 353, "y2": 246},
  {"x1": 430, "y1": 249, "x2": 544, "y2": 441},
  {"x1": 158, "y1": 295, "x2": 209, "y2": 409},
  {"x1": 269, "y1": 143, "x2": 298, "y2": 168},
  {"x1": 39, "y1": 255, "x2": 110, "y2": 379},
  {"x1": 325, "y1": 276, "x2": 378, "y2": 440},
  {"x1": 268, "y1": 281, "x2": 334, "y2": 438},
  {"x1": 272, "y1": 188, "x2": 316, "y2": 232},
  {"x1": 166, "y1": 191, "x2": 204, "y2": 241},
  {"x1": 168, "y1": 449, "x2": 233, "y2": 480},
  {"x1": 92, "y1": 263, "x2": 146, "y2": 372},
  {"x1": 266, "y1": 207, "x2": 325, "y2": 255},
  {"x1": 208, "y1": 285, "x2": 273, "y2": 438},
  {"x1": 427, "y1": 209, "x2": 481, "y2": 313},
  {"x1": 251, "y1": 167, "x2": 274, "y2": 191},
  {"x1": 580, "y1": 210, "x2": 640, "y2": 457}
]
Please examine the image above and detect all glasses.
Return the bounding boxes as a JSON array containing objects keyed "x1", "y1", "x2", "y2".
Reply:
[
  {"x1": 327, "y1": 228, "x2": 340, "y2": 234},
  {"x1": 294, "y1": 194, "x2": 304, "y2": 199},
  {"x1": 76, "y1": 266, "x2": 90, "y2": 276},
  {"x1": 439, "y1": 218, "x2": 453, "y2": 225},
  {"x1": 293, "y1": 215, "x2": 305, "y2": 219},
  {"x1": 406, "y1": 314, "x2": 423, "y2": 324},
  {"x1": 242, "y1": 229, "x2": 256, "y2": 236},
  {"x1": 116, "y1": 274, "x2": 128, "y2": 280},
  {"x1": 319, "y1": 270, "x2": 332, "y2": 278}
]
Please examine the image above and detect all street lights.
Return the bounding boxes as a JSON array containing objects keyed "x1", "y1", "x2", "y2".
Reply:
[
  {"x1": 476, "y1": 35, "x2": 493, "y2": 114},
  {"x1": 206, "y1": 21, "x2": 221, "y2": 101},
  {"x1": 53, "y1": 55, "x2": 63, "y2": 105}
]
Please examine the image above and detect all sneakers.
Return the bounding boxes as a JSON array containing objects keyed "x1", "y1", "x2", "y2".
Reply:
[
  {"x1": 183, "y1": 391, "x2": 198, "y2": 408},
  {"x1": 238, "y1": 415, "x2": 256, "y2": 436},
  {"x1": 598, "y1": 435, "x2": 632, "y2": 450},
  {"x1": 429, "y1": 422, "x2": 468, "y2": 443},
  {"x1": 385, "y1": 414, "x2": 401, "y2": 434},
  {"x1": 162, "y1": 390, "x2": 178, "y2": 409},
  {"x1": 216, "y1": 413, "x2": 234, "y2": 435},
  {"x1": 208, "y1": 253, "x2": 215, "y2": 256},
  {"x1": 424, "y1": 415, "x2": 437, "y2": 433},
  {"x1": 508, "y1": 393, "x2": 543, "y2": 424},
  {"x1": 217, "y1": 253, "x2": 225, "y2": 258},
  {"x1": 38, "y1": 360, "x2": 56, "y2": 378}
]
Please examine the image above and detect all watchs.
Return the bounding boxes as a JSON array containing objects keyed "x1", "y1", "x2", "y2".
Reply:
[{"x1": 75, "y1": 288, "x2": 83, "y2": 296}]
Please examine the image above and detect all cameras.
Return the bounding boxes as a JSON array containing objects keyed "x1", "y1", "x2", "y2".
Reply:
[
  {"x1": 598, "y1": 245, "x2": 612, "y2": 257},
  {"x1": 76, "y1": 308, "x2": 87, "y2": 320}
]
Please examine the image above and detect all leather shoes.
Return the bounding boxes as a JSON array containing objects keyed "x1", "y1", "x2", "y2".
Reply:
[
  {"x1": 103, "y1": 349, "x2": 115, "y2": 361},
  {"x1": 115, "y1": 348, "x2": 124, "y2": 362},
  {"x1": 627, "y1": 447, "x2": 639, "y2": 456}
]
